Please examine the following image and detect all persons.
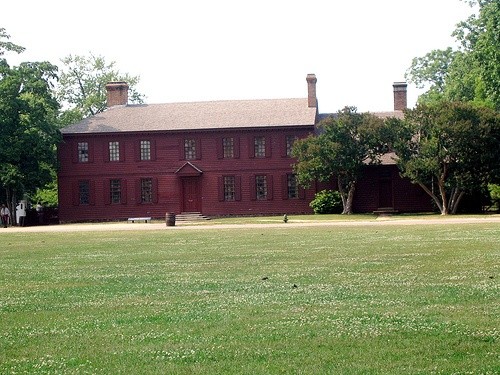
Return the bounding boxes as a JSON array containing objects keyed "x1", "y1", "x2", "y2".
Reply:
[{"x1": 0, "y1": 203, "x2": 11, "y2": 228}]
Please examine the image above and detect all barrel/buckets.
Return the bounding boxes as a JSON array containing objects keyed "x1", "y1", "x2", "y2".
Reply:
[{"x1": 166, "y1": 212, "x2": 175, "y2": 226}]
